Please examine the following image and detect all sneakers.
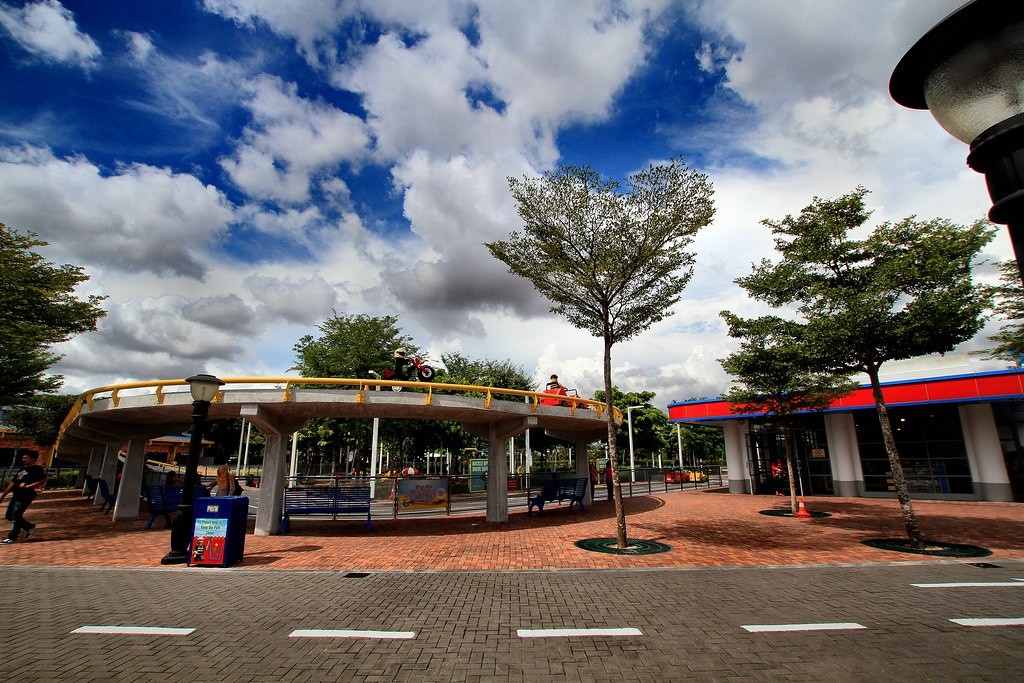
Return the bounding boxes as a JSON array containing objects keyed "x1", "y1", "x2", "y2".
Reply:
[
  {"x1": 24, "y1": 524, "x2": 36, "y2": 540},
  {"x1": 0, "y1": 538, "x2": 15, "y2": 544}
]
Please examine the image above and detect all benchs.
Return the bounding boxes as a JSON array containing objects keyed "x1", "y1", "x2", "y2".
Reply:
[
  {"x1": 147, "y1": 486, "x2": 203, "y2": 528},
  {"x1": 528, "y1": 479, "x2": 587, "y2": 515},
  {"x1": 282, "y1": 487, "x2": 371, "y2": 534},
  {"x1": 84, "y1": 475, "x2": 116, "y2": 514}
]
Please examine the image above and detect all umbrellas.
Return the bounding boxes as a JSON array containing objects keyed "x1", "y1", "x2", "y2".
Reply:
[{"x1": 401, "y1": 467, "x2": 419, "y2": 476}]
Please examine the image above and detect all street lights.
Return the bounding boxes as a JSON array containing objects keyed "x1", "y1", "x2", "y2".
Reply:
[
  {"x1": 626, "y1": 405, "x2": 647, "y2": 485},
  {"x1": 888, "y1": 0, "x2": 1023, "y2": 275},
  {"x1": 368, "y1": 370, "x2": 387, "y2": 504},
  {"x1": 159, "y1": 371, "x2": 229, "y2": 571}
]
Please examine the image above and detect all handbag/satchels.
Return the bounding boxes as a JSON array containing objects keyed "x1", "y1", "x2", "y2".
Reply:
[{"x1": 226, "y1": 473, "x2": 244, "y2": 496}]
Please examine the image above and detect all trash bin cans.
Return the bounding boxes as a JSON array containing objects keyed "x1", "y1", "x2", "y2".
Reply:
[{"x1": 188, "y1": 495, "x2": 250, "y2": 568}]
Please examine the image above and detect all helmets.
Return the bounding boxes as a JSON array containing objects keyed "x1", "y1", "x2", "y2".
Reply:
[{"x1": 550, "y1": 375, "x2": 558, "y2": 379}]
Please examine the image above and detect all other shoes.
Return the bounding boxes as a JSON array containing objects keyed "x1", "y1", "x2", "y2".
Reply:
[{"x1": 778, "y1": 492, "x2": 785, "y2": 496}]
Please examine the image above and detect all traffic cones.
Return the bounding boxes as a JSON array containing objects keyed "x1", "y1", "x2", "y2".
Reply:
[{"x1": 797, "y1": 497, "x2": 815, "y2": 520}]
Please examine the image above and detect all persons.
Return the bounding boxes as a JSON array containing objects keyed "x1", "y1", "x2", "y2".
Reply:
[
  {"x1": 165, "y1": 471, "x2": 184, "y2": 487},
  {"x1": 550, "y1": 374, "x2": 566, "y2": 390},
  {"x1": 527, "y1": 473, "x2": 561, "y2": 518},
  {"x1": 775, "y1": 458, "x2": 784, "y2": 478},
  {"x1": 394, "y1": 347, "x2": 414, "y2": 381},
  {"x1": 591, "y1": 466, "x2": 600, "y2": 482},
  {"x1": 600, "y1": 460, "x2": 615, "y2": 501},
  {"x1": 0, "y1": 451, "x2": 46, "y2": 543},
  {"x1": 206, "y1": 465, "x2": 235, "y2": 497},
  {"x1": 770, "y1": 460, "x2": 785, "y2": 496}
]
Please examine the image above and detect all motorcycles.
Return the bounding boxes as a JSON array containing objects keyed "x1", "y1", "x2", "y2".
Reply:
[{"x1": 383, "y1": 358, "x2": 436, "y2": 394}]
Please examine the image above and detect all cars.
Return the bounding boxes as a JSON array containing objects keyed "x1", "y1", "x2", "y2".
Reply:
[
  {"x1": 666, "y1": 469, "x2": 690, "y2": 485},
  {"x1": 541, "y1": 388, "x2": 587, "y2": 407},
  {"x1": 690, "y1": 471, "x2": 710, "y2": 483}
]
